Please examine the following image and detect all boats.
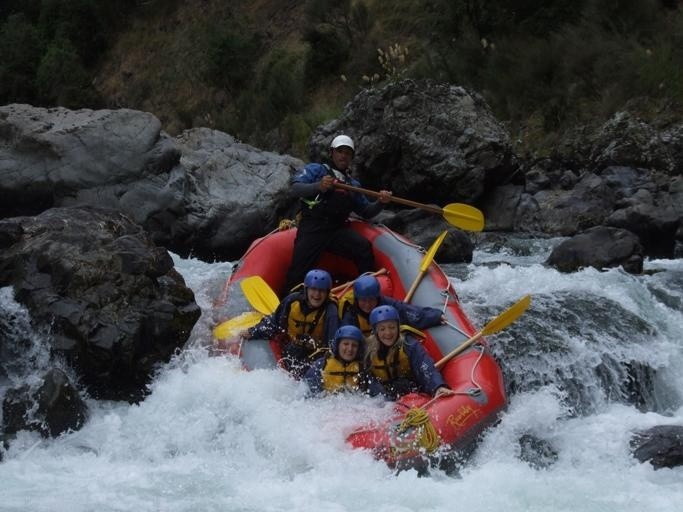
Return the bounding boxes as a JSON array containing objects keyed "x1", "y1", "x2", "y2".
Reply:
[{"x1": 212, "y1": 214, "x2": 510, "y2": 482}]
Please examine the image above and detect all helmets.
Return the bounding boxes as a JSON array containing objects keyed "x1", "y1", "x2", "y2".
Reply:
[
  {"x1": 354, "y1": 274, "x2": 381, "y2": 303},
  {"x1": 304, "y1": 268, "x2": 334, "y2": 299},
  {"x1": 369, "y1": 305, "x2": 401, "y2": 334},
  {"x1": 332, "y1": 325, "x2": 364, "y2": 359}
]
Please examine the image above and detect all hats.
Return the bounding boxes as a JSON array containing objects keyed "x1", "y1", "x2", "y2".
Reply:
[{"x1": 330, "y1": 134, "x2": 354, "y2": 152}]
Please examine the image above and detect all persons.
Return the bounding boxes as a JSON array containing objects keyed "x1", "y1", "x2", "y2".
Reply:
[
  {"x1": 282, "y1": 135, "x2": 393, "y2": 300},
  {"x1": 336, "y1": 274, "x2": 449, "y2": 345},
  {"x1": 357, "y1": 303, "x2": 456, "y2": 399},
  {"x1": 235, "y1": 267, "x2": 340, "y2": 379},
  {"x1": 303, "y1": 326, "x2": 388, "y2": 408}
]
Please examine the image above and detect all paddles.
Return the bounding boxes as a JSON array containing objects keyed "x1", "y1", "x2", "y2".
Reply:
[
  {"x1": 335, "y1": 180, "x2": 485, "y2": 234},
  {"x1": 434, "y1": 294, "x2": 530, "y2": 369},
  {"x1": 213, "y1": 267, "x2": 386, "y2": 343},
  {"x1": 402, "y1": 228, "x2": 448, "y2": 303}
]
